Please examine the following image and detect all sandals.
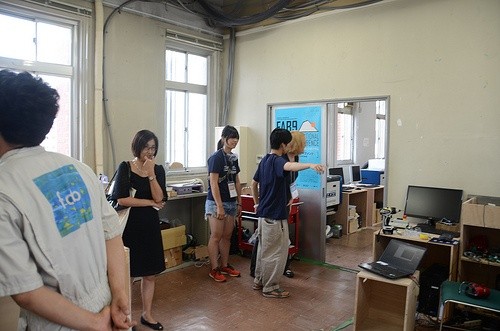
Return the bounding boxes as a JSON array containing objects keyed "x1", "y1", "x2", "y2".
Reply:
[
  {"x1": 263, "y1": 289, "x2": 289, "y2": 298},
  {"x1": 252, "y1": 283, "x2": 262, "y2": 290}
]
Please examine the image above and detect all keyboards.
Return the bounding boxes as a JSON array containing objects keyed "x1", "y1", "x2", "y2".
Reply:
[{"x1": 413, "y1": 226, "x2": 460, "y2": 238}]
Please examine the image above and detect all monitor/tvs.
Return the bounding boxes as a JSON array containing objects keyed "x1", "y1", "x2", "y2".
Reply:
[
  {"x1": 404, "y1": 186, "x2": 463, "y2": 225},
  {"x1": 329, "y1": 168, "x2": 344, "y2": 185},
  {"x1": 350, "y1": 165, "x2": 361, "y2": 184}
]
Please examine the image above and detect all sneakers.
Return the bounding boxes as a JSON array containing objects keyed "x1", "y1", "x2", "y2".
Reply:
[
  {"x1": 221, "y1": 263, "x2": 241, "y2": 277},
  {"x1": 209, "y1": 267, "x2": 226, "y2": 281}
]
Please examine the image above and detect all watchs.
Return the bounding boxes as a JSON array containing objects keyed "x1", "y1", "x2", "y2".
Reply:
[{"x1": 148, "y1": 175, "x2": 157, "y2": 181}]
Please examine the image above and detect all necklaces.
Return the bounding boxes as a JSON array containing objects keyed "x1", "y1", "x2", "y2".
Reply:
[{"x1": 132, "y1": 159, "x2": 149, "y2": 174}]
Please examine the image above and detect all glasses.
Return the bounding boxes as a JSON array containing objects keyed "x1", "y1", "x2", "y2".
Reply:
[{"x1": 141, "y1": 144, "x2": 157, "y2": 151}]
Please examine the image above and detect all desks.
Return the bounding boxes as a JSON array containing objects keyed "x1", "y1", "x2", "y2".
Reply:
[
  {"x1": 373, "y1": 229, "x2": 460, "y2": 281},
  {"x1": 167, "y1": 191, "x2": 208, "y2": 246},
  {"x1": 237, "y1": 196, "x2": 300, "y2": 259}
]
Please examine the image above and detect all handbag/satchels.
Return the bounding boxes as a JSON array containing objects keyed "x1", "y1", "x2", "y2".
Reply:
[{"x1": 104, "y1": 160, "x2": 131, "y2": 211}]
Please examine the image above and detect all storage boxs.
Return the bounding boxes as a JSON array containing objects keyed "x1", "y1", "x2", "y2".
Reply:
[
  {"x1": 326, "y1": 175, "x2": 343, "y2": 207},
  {"x1": 360, "y1": 169, "x2": 384, "y2": 185}
]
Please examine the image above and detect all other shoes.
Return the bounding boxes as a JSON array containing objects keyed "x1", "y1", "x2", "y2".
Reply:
[
  {"x1": 140, "y1": 316, "x2": 163, "y2": 330},
  {"x1": 284, "y1": 269, "x2": 295, "y2": 278}
]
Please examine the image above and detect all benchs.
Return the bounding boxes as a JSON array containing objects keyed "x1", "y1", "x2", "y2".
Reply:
[{"x1": 437, "y1": 281, "x2": 500, "y2": 331}]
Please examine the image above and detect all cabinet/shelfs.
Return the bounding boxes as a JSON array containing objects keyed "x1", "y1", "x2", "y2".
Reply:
[{"x1": 335, "y1": 181, "x2": 384, "y2": 234}]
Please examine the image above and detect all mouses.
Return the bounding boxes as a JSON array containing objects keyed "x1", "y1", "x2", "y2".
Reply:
[{"x1": 355, "y1": 188, "x2": 361, "y2": 190}]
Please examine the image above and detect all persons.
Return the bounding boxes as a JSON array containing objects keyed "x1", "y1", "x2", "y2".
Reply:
[
  {"x1": 0, "y1": 69, "x2": 139, "y2": 331},
  {"x1": 203, "y1": 124, "x2": 242, "y2": 283},
  {"x1": 104, "y1": 129, "x2": 168, "y2": 331},
  {"x1": 249, "y1": 127, "x2": 327, "y2": 298}
]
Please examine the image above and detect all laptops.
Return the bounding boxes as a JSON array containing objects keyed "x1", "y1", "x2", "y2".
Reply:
[{"x1": 358, "y1": 239, "x2": 428, "y2": 279}]
